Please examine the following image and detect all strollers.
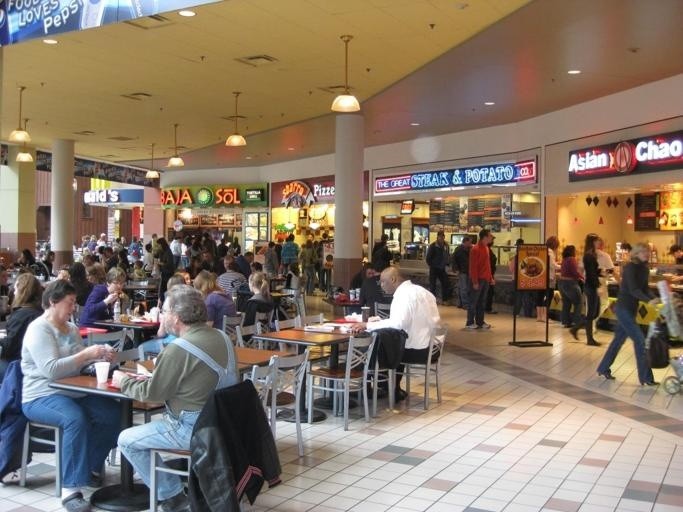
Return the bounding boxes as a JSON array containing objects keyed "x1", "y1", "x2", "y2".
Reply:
[{"x1": 655, "y1": 279, "x2": 683, "y2": 396}]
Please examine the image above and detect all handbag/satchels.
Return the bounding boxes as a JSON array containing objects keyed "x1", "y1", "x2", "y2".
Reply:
[{"x1": 646, "y1": 317, "x2": 670, "y2": 368}]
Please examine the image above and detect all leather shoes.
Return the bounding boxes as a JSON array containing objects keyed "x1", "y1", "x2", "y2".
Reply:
[
  {"x1": 587, "y1": 338, "x2": 600, "y2": 345},
  {"x1": 570, "y1": 327, "x2": 578, "y2": 340}
]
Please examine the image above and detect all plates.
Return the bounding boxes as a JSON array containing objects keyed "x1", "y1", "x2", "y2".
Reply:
[
  {"x1": 658, "y1": 191, "x2": 681, "y2": 227},
  {"x1": 519, "y1": 257, "x2": 545, "y2": 279}
]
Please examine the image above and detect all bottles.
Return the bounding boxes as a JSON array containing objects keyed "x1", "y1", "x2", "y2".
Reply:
[{"x1": 114, "y1": 298, "x2": 121, "y2": 322}]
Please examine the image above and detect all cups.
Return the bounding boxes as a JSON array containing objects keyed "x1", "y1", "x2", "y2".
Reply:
[
  {"x1": 614, "y1": 242, "x2": 627, "y2": 261},
  {"x1": 361, "y1": 307, "x2": 370, "y2": 322},
  {"x1": 150, "y1": 308, "x2": 160, "y2": 323},
  {"x1": 348, "y1": 288, "x2": 360, "y2": 302},
  {"x1": 94, "y1": 361, "x2": 111, "y2": 383}
]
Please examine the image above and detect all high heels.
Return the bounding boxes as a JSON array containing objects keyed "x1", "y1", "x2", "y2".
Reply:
[
  {"x1": 597, "y1": 369, "x2": 615, "y2": 380},
  {"x1": 640, "y1": 381, "x2": 659, "y2": 386}
]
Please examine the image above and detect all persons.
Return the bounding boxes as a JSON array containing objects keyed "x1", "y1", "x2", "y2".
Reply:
[
  {"x1": 349, "y1": 262, "x2": 394, "y2": 319},
  {"x1": 371, "y1": 234, "x2": 393, "y2": 272},
  {"x1": 508, "y1": 233, "x2": 620, "y2": 346},
  {"x1": 425, "y1": 228, "x2": 497, "y2": 328},
  {"x1": 118, "y1": 285, "x2": 239, "y2": 512},
  {"x1": 353, "y1": 267, "x2": 440, "y2": 400},
  {"x1": 596, "y1": 241, "x2": 660, "y2": 386},
  {"x1": 20, "y1": 279, "x2": 122, "y2": 512},
  {"x1": 1, "y1": 228, "x2": 333, "y2": 363},
  {"x1": 667, "y1": 244, "x2": 683, "y2": 284}
]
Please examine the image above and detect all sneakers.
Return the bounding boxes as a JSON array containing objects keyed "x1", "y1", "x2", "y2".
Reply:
[
  {"x1": 480, "y1": 323, "x2": 491, "y2": 329},
  {"x1": 466, "y1": 324, "x2": 479, "y2": 329}
]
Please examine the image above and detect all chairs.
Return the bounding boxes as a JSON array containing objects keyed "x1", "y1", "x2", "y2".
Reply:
[{"x1": 0, "y1": 230, "x2": 448, "y2": 510}]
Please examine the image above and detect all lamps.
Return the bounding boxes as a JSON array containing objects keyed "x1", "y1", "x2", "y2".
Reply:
[
  {"x1": 144, "y1": 142, "x2": 159, "y2": 180},
  {"x1": 329, "y1": 32, "x2": 363, "y2": 114},
  {"x1": 165, "y1": 123, "x2": 185, "y2": 169},
  {"x1": 14, "y1": 118, "x2": 33, "y2": 164},
  {"x1": 9, "y1": 83, "x2": 31, "y2": 142},
  {"x1": 222, "y1": 89, "x2": 249, "y2": 150}
]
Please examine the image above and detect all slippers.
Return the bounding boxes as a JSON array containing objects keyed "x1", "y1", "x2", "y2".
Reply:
[{"x1": 62, "y1": 491, "x2": 91, "y2": 511}]
